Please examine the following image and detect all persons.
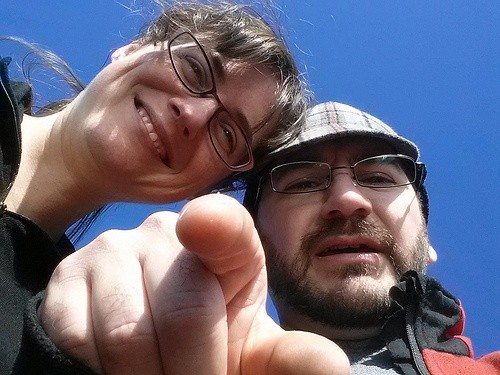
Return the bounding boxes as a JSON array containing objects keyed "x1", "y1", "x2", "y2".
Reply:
[
  {"x1": 0, "y1": 0, "x2": 310, "y2": 374},
  {"x1": 25, "y1": 101, "x2": 500, "y2": 375}
]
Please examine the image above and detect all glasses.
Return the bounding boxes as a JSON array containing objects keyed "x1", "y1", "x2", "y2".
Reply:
[
  {"x1": 168, "y1": 27, "x2": 254, "y2": 171},
  {"x1": 256, "y1": 153, "x2": 426, "y2": 203}
]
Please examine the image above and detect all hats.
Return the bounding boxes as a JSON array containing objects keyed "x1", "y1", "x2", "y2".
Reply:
[{"x1": 269, "y1": 102, "x2": 420, "y2": 163}]
